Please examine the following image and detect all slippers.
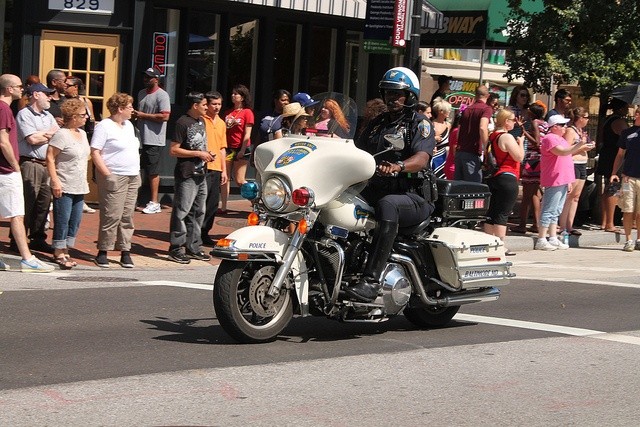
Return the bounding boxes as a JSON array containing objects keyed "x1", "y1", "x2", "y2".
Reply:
[
  {"x1": 558, "y1": 231, "x2": 572, "y2": 235},
  {"x1": 570, "y1": 230, "x2": 582, "y2": 235},
  {"x1": 503, "y1": 247, "x2": 516, "y2": 256},
  {"x1": 605, "y1": 228, "x2": 623, "y2": 233}
]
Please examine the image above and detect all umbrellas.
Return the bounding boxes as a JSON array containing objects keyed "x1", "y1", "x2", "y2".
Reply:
[{"x1": 607, "y1": 81, "x2": 640, "y2": 106}]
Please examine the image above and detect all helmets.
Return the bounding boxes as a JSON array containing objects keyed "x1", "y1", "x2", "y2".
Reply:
[{"x1": 380, "y1": 67, "x2": 421, "y2": 105}]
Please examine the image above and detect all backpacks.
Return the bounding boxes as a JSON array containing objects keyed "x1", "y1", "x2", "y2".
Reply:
[{"x1": 482, "y1": 132, "x2": 509, "y2": 179}]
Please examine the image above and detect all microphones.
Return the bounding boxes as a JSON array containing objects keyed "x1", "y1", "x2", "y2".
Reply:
[{"x1": 394, "y1": 100, "x2": 414, "y2": 108}]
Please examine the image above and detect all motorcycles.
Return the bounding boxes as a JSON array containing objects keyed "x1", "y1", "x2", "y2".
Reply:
[{"x1": 209, "y1": 92, "x2": 516, "y2": 342}]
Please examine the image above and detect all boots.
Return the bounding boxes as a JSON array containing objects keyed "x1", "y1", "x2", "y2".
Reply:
[{"x1": 347, "y1": 220, "x2": 399, "y2": 303}]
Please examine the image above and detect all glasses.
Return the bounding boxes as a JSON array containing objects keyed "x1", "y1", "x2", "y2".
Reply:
[
  {"x1": 520, "y1": 93, "x2": 529, "y2": 98},
  {"x1": 67, "y1": 84, "x2": 76, "y2": 87},
  {"x1": 75, "y1": 112, "x2": 87, "y2": 117},
  {"x1": 10, "y1": 85, "x2": 23, "y2": 88},
  {"x1": 555, "y1": 123, "x2": 567, "y2": 126},
  {"x1": 120, "y1": 104, "x2": 132, "y2": 110},
  {"x1": 583, "y1": 116, "x2": 590, "y2": 119}
]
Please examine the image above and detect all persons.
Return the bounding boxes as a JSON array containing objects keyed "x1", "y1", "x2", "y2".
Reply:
[
  {"x1": 609, "y1": 106, "x2": 640, "y2": 251},
  {"x1": 130, "y1": 67, "x2": 172, "y2": 214},
  {"x1": 345, "y1": 65, "x2": 436, "y2": 303},
  {"x1": 597, "y1": 97, "x2": 630, "y2": 234},
  {"x1": 0, "y1": 68, "x2": 94, "y2": 271},
  {"x1": 558, "y1": 107, "x2": 596, "y2": 235},
  {"x1": 89, "y1": 92, "x2": 143, "y2": 269},
  {"x1": 249, "y1": 88, "x2": 292, "y2": 168},
  {"x1": 200, "y1": 90, "x2": 229, "y2": 248},
  {"x1": 284, "y1": 92, "x2": 348, "y2": 137},
  {"x1": 545, "y1": 88, "x2": 572, "y2": 122},
  {"x1": 415, "y1": 100, "x2": 433, "y2": 156},
  {"x1": 168, "y1": 91, "x2": 211, "y2": 264},
  {"x1": 432, "y1": 74, "x2": 542, "y2": 255},
  {"x1": 534, "y1": 114, "x2": 588, "y2": 251},
  {"x1": 215, "y1": 83, "x2": 254, "y2": 215}
]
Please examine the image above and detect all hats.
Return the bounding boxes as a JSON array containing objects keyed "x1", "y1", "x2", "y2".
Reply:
[
  {"x1": 293, "y1": 93, "x2": 320, "y2": 107},
  {"x1": 144, "y1": 68, "x2": 160, "y2": 78},
  {"x1": 283, "y1": 102, "x2": 310, "y2": 117},
  {"x1": 28, "y1": 82, "x2": 56, "y2": 95},
  {"x1": 548, "y1": 115, "x2": 571, "y2": 127}
]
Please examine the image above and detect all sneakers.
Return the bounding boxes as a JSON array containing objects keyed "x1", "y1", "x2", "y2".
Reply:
[
  {"x1": 30, "y1": 241, "x2": 51, "y2": 251},
  {"x1": 510, "y1": 226, "x2": 525, "y2": 233},
  {"x1": 202, "y1": 239, "x2": 214, "y2": 245},
  {"x1": 635, "y1": 241, "x2": 640, "y2": 250},
  {"x1": 21, "y1": 255, "x2": 55, "y2": 273},
  {"x1": 549, "y1": 238, "x2": 569, "y2": 250},
  {"x1": 216, "y1": 208, "x2": 227, "y2": 215},
  {"x1": 185, "y1": 251, "x2": 211, "y2": 260},
  {"x1": 120, "y1": 256, "x2": 134, "y2": 267},
  {"x1": 534, "y1": 240, "x2": 557, "y2": 250},
  {"x1": 54, "y1": 258, "x2": 72, "y2": 268},
  {"x1": 82, "y1": 202, "x2": 96, "y2": 213},
  {"x1": 94, "y1": 256, "x2": 109, "y2": 267},
  {"x1": 0, "y1": 259, "x2": 10, "y2": 270},
  {"x1": 168, "y1": 252, "x2": 191, "y2": 264},
  {"x1": 528, "y1": 226, "x2": 538, "y2": 233},
  {"x1": 65, "y1": 256, "x2": 78, "y2": 266},
  {"x1": 10, "y1": 240, "x2": 19, "y2": 252},
  {"x1": 142, "y1": 201, "x2": 161, "y2": 214},
  {"x1": 44, "y1": 216, "x2": 50, "y2": 230},
  {"x1": 624, "y1": 241, "x2": 634, "y2": 251}
]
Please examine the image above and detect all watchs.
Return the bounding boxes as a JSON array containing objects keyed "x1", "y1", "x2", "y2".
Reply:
[{"x1": 396, "y1": 160, "x2": 405, "y2": 172}]
